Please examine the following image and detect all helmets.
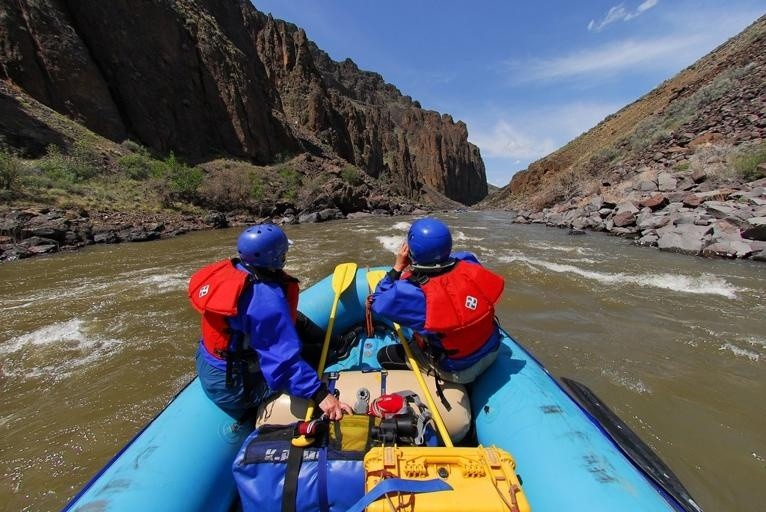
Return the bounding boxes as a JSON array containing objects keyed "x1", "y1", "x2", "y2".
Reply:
[
  {"x1": 407, "y1": 217, "x2": 453, "y2": 265},
  {"x1": 237, "y1": 223, "x2": 289, "y2": 273}
]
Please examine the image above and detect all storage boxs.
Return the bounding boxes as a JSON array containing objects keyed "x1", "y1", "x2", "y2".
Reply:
[{"x1": 364, "y1": 443, "x2": 531, "y2": 512}]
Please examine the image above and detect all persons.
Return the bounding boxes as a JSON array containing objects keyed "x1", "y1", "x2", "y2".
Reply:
[
  {"x1": 187, "y1": 224, "x2": 358, "y2": 421},
  {"x1": 371, "y1": 217, "x2": 506, "y2": 385}
]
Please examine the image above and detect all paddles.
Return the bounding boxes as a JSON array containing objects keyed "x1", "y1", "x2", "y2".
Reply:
[
  {"x1": 291, "y1": 263, "x2": 357, "y2": 448},
  {"x1": 366, "y1": 270, "x2": 454, "y2": 448}
]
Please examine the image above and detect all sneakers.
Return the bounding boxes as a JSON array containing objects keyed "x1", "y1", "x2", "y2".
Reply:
[{"x1": 335, "y1": 331, "x2": 358, "y2": 360}]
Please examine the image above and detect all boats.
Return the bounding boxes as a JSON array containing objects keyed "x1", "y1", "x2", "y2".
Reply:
[{"x1": 61, "y1": 266, "x2": 706, "y2": 512}]
